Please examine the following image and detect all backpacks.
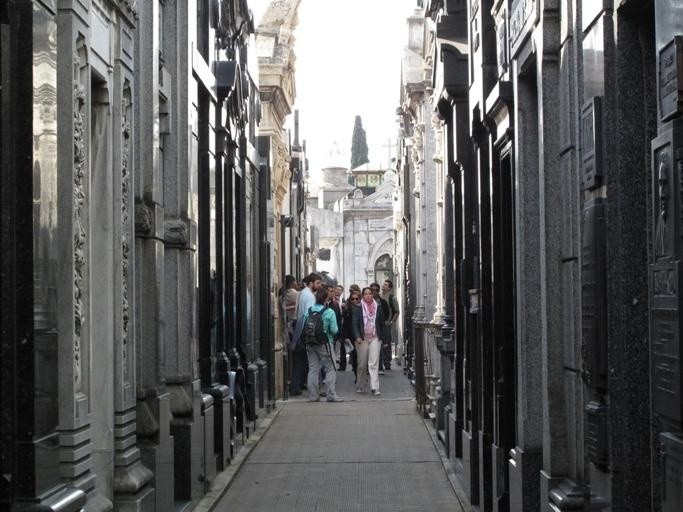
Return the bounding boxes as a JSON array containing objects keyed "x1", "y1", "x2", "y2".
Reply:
[{"x1": 305, "y1": 306, "x2": 329, "y2": 345}]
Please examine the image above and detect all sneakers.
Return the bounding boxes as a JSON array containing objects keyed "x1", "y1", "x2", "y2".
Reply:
[
  {"x1": 290, "y1": 391, "x2": 302, "y2": 395},
  {"x1": 320, "y1": 392, "x2": 326, "y2": 397},
  {"x1": 297, "y1": 385, "x2": 306, "y2": 390},
  {"x1": 356, "y1": 388, "x2": 362, "y2": 392},
  {"x1": 353, "y1": 364, "x2": 390, "y2": 384},
  {"x1": 328, "y1": 396, "x2": 344, "y2": 402},
  {"x1": 338, "y1": 367, "x2": 344, "y2": 370},
  {"x1": 371, "y1": 390, "x2": 380, "y2": 395}
]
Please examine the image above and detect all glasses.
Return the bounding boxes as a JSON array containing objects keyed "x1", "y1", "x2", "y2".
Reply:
[{"x1": 351, "y1": 298, "x2": 358, "y2": 301}]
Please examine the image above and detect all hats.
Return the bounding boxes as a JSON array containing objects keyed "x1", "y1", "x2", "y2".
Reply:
[{"x1": 350, "y1": 284, "x2": 360, "y2": 292}]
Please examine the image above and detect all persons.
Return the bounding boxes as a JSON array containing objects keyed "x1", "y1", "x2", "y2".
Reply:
[{"x1": 283, "y1": 274, "x2": 400, "y2": 402}]
